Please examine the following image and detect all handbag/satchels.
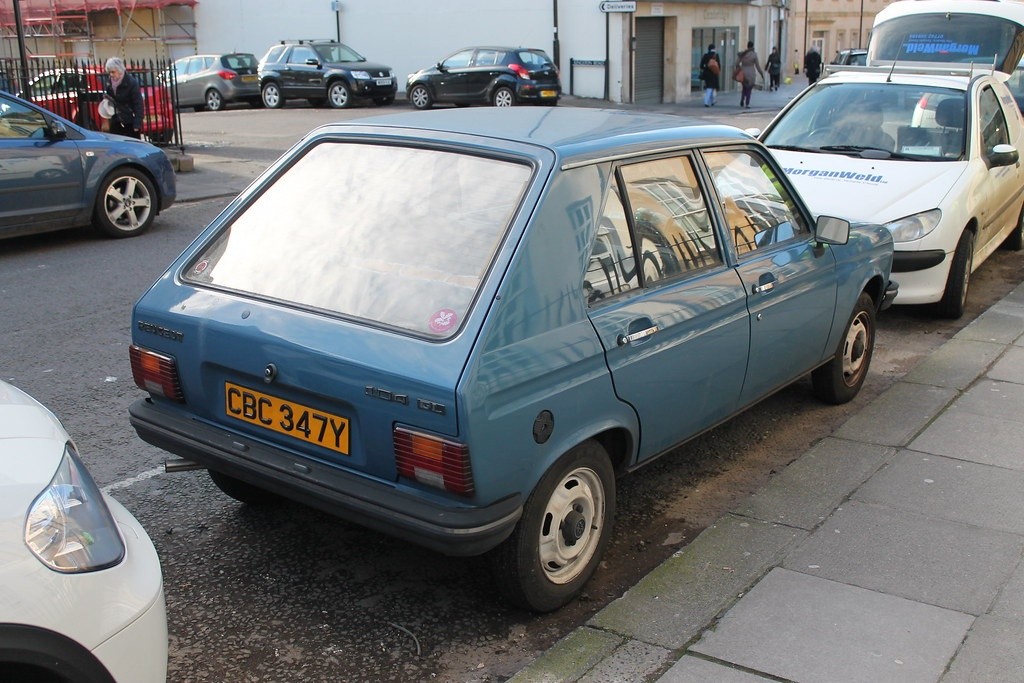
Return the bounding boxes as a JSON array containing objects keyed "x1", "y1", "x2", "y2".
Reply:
[
  {"x1": 733, "y1": 65, "x2": 744, "y2": 82},
  {"x1": 116, "y1": 104, "x2": 137, "y2": 124},
  {"x1": 707, "y1": 52, "x2": 720, "y2": 75},
  {"x1": 773, "y1": 63, "x2": 779, "y2": 69}
]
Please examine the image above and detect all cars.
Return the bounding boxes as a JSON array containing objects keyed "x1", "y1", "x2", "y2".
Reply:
[
  {"x1": 14, "y1": 65, "x2": 174, "y2": 148},
  {"x1": 156, "y1": 53, "x2": 260, "y2": 111},
  {"x1": 713, "y1": 0, "x2": 1024, "y2": 319},
  {"x1": 0, "y1": 91, "x2": 177, "y2": 239},
  {"x1": 912, "y1": 58, "x2": 1024, "y2": 138},
  {"x1": 1, "y1": 379, "x2": 169, "y2": 683},
  {"x1": 406, "y1": 46, "x2": 562, "y2": 111},
  {"x1": 831, "y1": 49, "x2": 869, "y2": 65},
  {"x1": 125, "y1": 105, "x2": 900, "y2": 618}
]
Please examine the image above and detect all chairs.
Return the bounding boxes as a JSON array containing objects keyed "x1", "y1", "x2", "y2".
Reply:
[
  {"x1": 919, "y1": 97, "x2": 966, "y2": 155},
  {"x1": 828, "y1": 101, "x2": 897, "y2": 154}
]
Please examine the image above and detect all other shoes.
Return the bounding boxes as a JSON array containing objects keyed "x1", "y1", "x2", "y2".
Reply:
[
  {"x1": 770, "y1": 88, "x2": 773, "y2": 91},
  {"x1": 740, "y1": 104, "x2": 744, "y2": 107},
  {"x1": 705, "y1": 104, "x2": 709, "y2": 107},
  {"x1": 711, "y1": 103, "x2": 716, "y2": 106},
  {"x1": 746, "y1": 106, "x2": 751, "y2": 108},
  {"x1": 775, "y1": 87, "x2": 777, "y2": 91}
]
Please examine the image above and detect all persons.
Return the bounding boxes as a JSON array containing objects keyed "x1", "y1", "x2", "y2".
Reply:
[
  {"x1": 764, "y1": 47, "x2": 781, "y2": 92},
  {"x1": 804, "y1": 46, "x2": 822, "y2": 86},
  {"x1": 736, "y1": 41, "x2": 763, "y2": 108},
  {"x1": 105, "y1": 57, "x2": 144, "y2": 140},
  {"x1": 698, "y1": 44, "x2": 721, "y2": 107},
  {"x1": 793, "y1": 50, "x2": 799, "y2": 74}
]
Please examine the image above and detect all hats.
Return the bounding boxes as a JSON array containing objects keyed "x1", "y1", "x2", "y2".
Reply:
[
  {"x1": 97, "y1": 98, "x2": 116, "y2": 120},
  {"x1": 809, "y1": 44, "x2": 821, "y2": 53}
]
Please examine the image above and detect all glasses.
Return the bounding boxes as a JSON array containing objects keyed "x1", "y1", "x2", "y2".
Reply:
[{"x1": 108, "y1": 69, "x2": 119, "y2": 73}]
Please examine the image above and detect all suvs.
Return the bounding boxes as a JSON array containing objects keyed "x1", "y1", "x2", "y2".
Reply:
[{"x1": 257, "y1": 39, "x2": 399, "y2": 110}]
